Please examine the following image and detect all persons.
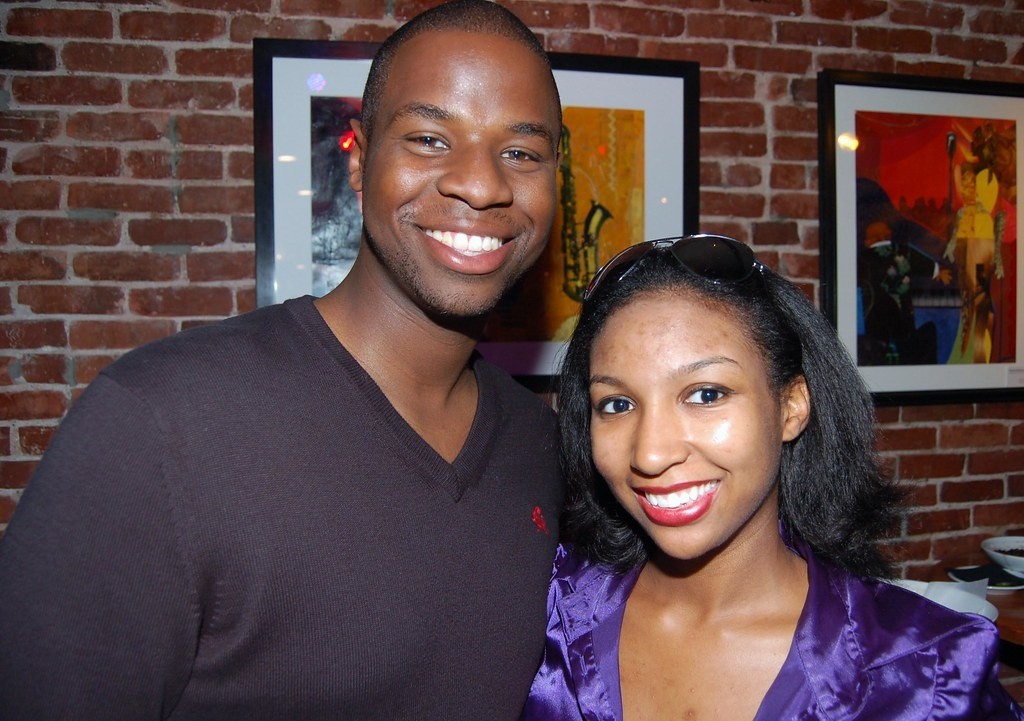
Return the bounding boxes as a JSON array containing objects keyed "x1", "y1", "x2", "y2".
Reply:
[
  {"x1": 0, "y1": 1, "x2": 561, "y2": 720},
  {"x1": 529, "y1": 235, "x2": 1023, "y2": 721}
]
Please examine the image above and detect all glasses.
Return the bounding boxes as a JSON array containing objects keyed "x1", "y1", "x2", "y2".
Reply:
[{"x1": 582, "y1": 234, "x2": 772, "y2": 309}]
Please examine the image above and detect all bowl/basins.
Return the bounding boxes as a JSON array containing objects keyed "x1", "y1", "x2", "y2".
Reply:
[{"x1": 980, "y1": 536, "x2": 1024, "y2": 578}]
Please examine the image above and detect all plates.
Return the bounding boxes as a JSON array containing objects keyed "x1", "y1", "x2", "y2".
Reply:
[
  {"x1": 948, "y1": 564, "x2": 1024, "y2": 595},
  {"x1": 878, "y1": 579, "x2": 998, "y2": 623}
]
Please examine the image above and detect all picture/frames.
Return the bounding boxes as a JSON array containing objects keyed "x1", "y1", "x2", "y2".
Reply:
[
  {"x1": 816, "y1": 67, "x2": 1024, "y2": 408},
  {"x1": 252, "y1": 37, "x2": 699, "y2": 393}
]
